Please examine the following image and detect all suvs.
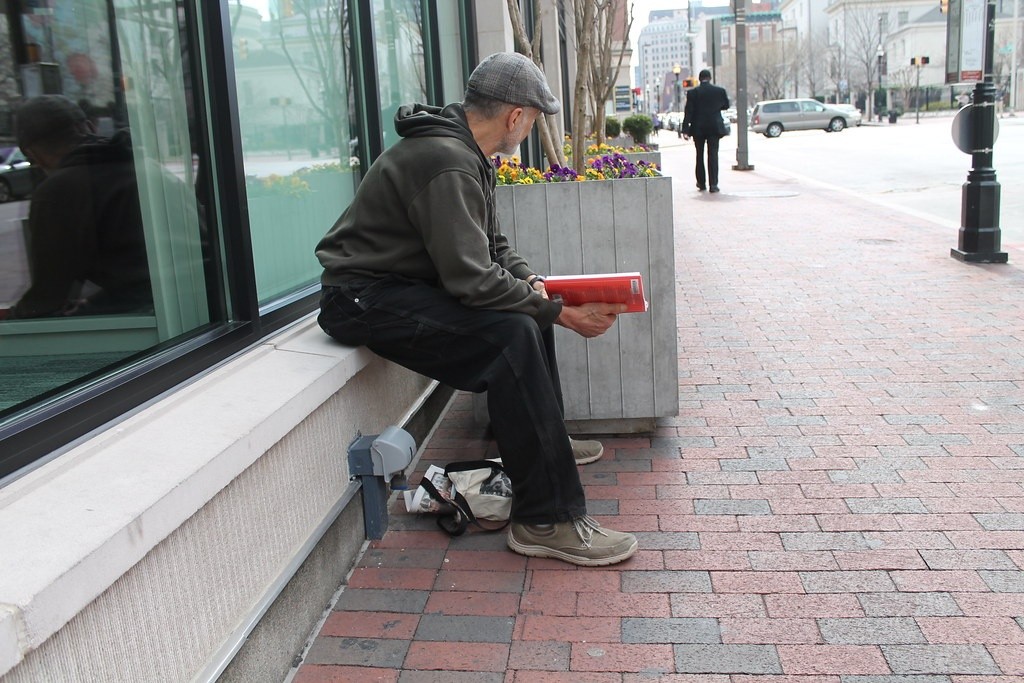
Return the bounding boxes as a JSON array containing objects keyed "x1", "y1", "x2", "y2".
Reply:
[{"x1": 751, "y1": 98, "x2": 857, "y2": 139}]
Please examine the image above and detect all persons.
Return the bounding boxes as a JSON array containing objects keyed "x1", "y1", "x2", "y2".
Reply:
[
  {"x1": 652, "y1": 114, "x2": 659, "y2": 137},
  {"x1": 5, "y1": 96, "x2": 202, "y2": 320},
  {"x1": 682, "y1": 70, "x2": 730, "y2": 193},
  {"x1": 315, "y1": 54, "x2": 638, "y2": 565}
]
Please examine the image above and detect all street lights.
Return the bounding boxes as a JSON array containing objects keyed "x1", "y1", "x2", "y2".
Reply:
[
  {"x1": 876, "y1": 44, "x2": 884, "y2": 122},
  {"x1": 673, "y1": 63, "x2": 683, "y2": 139},
  {"x1": 655, "y1": 78, "x2": 661, "y2": 114},
  {"x1": 641, "y1": 43, "x2": 652, "y2": 122},
  {"x1": 646, "y1": 79, "x2": 651, "y2": 115}
]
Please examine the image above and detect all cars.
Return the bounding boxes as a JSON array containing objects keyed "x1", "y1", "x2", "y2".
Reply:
[
  {"x1": 835, "y1": 103, "x2": 861, "y2": 125},
  {"x1": 658, "y1": 107, "x2": 751, "y2": 138}
]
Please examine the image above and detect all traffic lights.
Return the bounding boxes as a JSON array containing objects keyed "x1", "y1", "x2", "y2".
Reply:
[
  {"x1": 939, "y1": 0, "x2": 948, "y2": 14},
  {"x1": 911, "y1": 56, "x2": 920, "y2": 66},
  {"x1": 681, "y1": 79, "x2": 693, "y2": 89},
  {"x1": 921, "y1": 57, "x2": 930, "y2": 65}
]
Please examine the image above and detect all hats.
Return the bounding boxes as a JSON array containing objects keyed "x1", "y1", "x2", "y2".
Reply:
[
  {"x1": 466, "y1": 52, "x2": 560, "y2": 115},
  {"x1": 15, "y1": 94, "x2": 88, "y2": 149}
]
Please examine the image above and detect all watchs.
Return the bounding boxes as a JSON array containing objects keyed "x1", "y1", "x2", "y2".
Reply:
[{"x1": 528, "y1": 274, "x2": 546, "y2": 291}]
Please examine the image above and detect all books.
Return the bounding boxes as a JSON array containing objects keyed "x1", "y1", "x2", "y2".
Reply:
[{"x1": 543, "y1": 272, "x2": 648, "y2": 314}]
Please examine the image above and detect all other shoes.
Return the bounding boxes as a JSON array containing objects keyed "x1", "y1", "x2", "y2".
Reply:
[
  {"x1": 710, "y1": 185, "x2": 719, "y2": 192},
  {"x1": 696, "y1": 183, "x2": 706, "y2": 190}
]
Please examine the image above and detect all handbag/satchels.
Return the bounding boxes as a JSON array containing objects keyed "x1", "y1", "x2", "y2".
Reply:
[{"x1": 419, "y1": 457, "x2": 515, "y2": 536}]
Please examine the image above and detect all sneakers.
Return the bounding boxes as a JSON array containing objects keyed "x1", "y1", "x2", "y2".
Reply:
[
  {"x1": 567, "y1": 435, "x2": 603, "y2": 465},
  {"x1": 506, "y1": 513, "x2": 638, "y2": 566}
]
